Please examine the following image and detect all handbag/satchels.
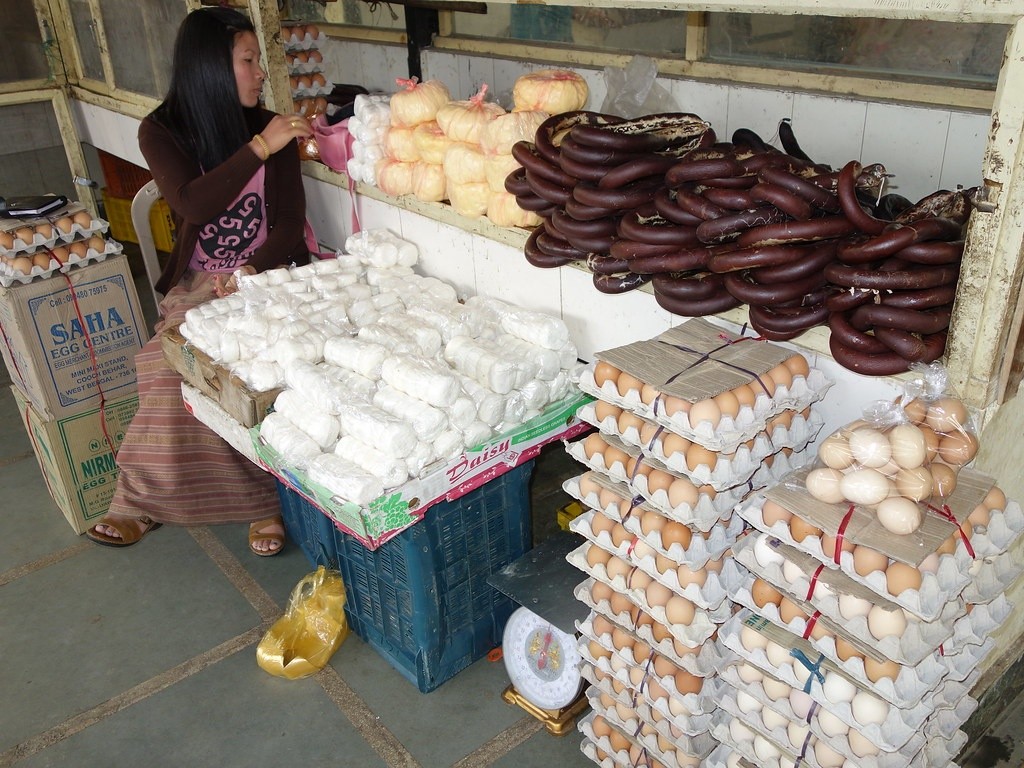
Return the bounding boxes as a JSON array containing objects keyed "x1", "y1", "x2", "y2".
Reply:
[{"x1": 304, "y1": 113, "x2": 361, "y2": 260}]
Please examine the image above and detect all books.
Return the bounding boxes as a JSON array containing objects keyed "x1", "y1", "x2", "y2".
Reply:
[{"x1": 0, "y1": 195, "x2": 68, "y2": 217}]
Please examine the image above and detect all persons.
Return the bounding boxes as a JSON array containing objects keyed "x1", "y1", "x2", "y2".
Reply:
[{"x1": 85, "y1": 6, "x2": 315, "y2": 558}]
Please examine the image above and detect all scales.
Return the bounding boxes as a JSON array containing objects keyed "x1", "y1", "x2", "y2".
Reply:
[{"x1": 484, "y1": 542, "x2": 591, "y2": 738}]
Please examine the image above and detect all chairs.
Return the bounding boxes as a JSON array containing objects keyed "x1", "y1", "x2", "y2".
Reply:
[{"x1": 129, "y1": 179, "x2": 165, "y2": 317}]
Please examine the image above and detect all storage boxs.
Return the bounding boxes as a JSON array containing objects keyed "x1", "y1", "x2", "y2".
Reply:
[
  {"x1": 159, "y1": 321, "x2": 598, "y2": 694},
  {"x1": 0, "y1": 251, "x2": 151, "y2": 422},
  {"x1": 97, "y1": 146, "x2": 153, "y2": 199},
  {"x1": 8, "y1": 384, "x2": 140, "y2": 537},
  {"x1": 101, "y1": 185, "x2": 174, "y2": 253}
]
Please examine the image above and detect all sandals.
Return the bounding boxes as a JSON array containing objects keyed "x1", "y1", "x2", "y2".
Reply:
[
  {"x1": 85, "y1": 509, "x2": 163, "y2": 548},
  {"x1": 248, "y1": 517, "x2": 283, "y2": 558}
]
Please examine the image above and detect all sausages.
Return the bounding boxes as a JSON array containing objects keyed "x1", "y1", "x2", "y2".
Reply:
[{"x1": 502, "y1": 111, "x2": 972, "y2": 377}]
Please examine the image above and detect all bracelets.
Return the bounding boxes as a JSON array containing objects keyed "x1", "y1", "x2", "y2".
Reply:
[{"x1": 253, "y1": 134, "x2": 269, "y2": 160}]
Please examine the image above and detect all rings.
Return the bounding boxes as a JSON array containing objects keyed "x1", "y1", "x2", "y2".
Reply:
[{"x1": 291, "y1": 120, "x2": 297, "y2": 128}]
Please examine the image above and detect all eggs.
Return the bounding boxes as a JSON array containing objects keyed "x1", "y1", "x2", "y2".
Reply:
[
  {"x1": 0, "y1": 211, "x2": 105, "y2": 275},
  {"x1": 280, "y1": 24, "x2": 328, "y2": 116},
  {"x1": 586, "y1": 357, "x2": 1008, "y2": 768}
]
